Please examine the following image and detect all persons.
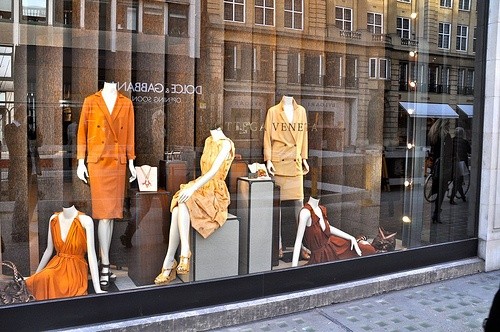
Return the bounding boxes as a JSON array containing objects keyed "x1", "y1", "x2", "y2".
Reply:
[
  {"x1": 156, "y1": 127, "x2": 235, "y2": 285},
  {"x1": 76, "y1": 82, "x2": 137, "y2": 289},
  {"x1": 427, "y1": 119, "x2": 467, "y2": 225},
  {"x1": 264, "y1": 96, "x2": 310, "y2": 260},
  {"x1": 22, "y1": 204, "x2": 107, "y2": 301},
  {"x1": 7, "y1": 106, "x2": 173, "y2": 250},
  {"x1": 291, "y1": 194, "x2": 376, "y2": 267}
]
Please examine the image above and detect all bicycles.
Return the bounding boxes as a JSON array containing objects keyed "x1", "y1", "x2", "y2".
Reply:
[{"x1": 423, "y1": 149, "x2": 470, "y2": 203}]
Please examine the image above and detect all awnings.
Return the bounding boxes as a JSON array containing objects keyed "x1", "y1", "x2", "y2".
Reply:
[{"x1": 399, "y1": 101, "x2": 473, "y2": 118}]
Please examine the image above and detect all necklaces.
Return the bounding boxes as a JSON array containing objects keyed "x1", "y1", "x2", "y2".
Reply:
[{"x1": 140, "y1": 167, "x2": 154, "y2": 187}]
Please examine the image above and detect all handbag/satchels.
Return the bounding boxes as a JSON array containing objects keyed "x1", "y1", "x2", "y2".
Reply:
[
  {"x1": 371, "y1": 226, "x2": 397, "y2": 251},
  {"x1": 1, "y1": 260, "x2": 36, "y2": 305}
]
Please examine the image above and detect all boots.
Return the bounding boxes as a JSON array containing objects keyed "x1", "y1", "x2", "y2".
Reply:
[{"x1": 432, "y1": 208, "x2": 442, "y2": 224}]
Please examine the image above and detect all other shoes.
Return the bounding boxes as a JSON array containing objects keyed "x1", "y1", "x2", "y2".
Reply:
[
  {"x1": 450, "y1": 201, "x2": 457, "y2": 206},
  {"x1": 121, "y1": 235, "x2": 133, "y2": 249}
]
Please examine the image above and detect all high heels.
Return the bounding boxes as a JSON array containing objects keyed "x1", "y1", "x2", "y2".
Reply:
[
  {"x1": 177, "y1": 251, "x2": 192, "y2": 274},
  {"x1": 109, "y1": 271, "x2": 116, "y2": 282},
  {"x1": 154, "y1": 258, "x2": 177, "y2": 285},
  {"x1": 100, "y1": 264, "x2": 111, "y2": 291}
]
